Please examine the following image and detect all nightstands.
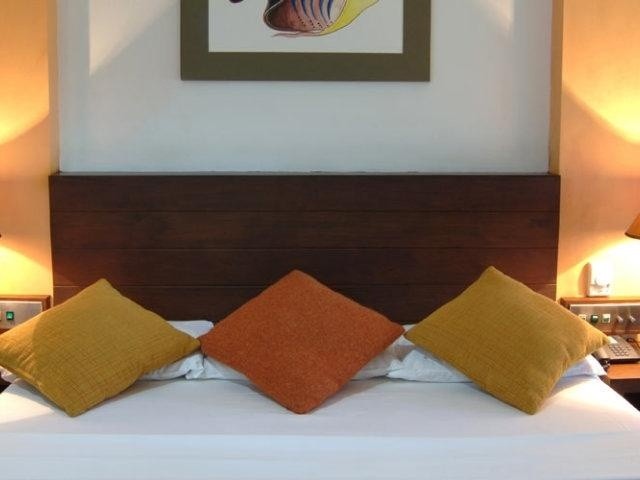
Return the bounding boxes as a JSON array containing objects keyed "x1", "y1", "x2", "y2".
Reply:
[{"x1": 561, "y1": 297, "x2": 640, "y2": 395}]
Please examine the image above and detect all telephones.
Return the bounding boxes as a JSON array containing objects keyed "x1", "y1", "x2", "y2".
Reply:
[{"x1": 593, "y1": 334, "x2": 640, "y2": 364}]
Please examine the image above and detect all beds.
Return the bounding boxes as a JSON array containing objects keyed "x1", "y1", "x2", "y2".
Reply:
[{"x1": 0, "y1": 175, "x2": 640, "y2": 480}]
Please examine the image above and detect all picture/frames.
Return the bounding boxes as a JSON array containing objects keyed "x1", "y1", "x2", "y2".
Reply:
[{"x1": 182, "y1": 0, "x2": 430, "y2": 80}]
